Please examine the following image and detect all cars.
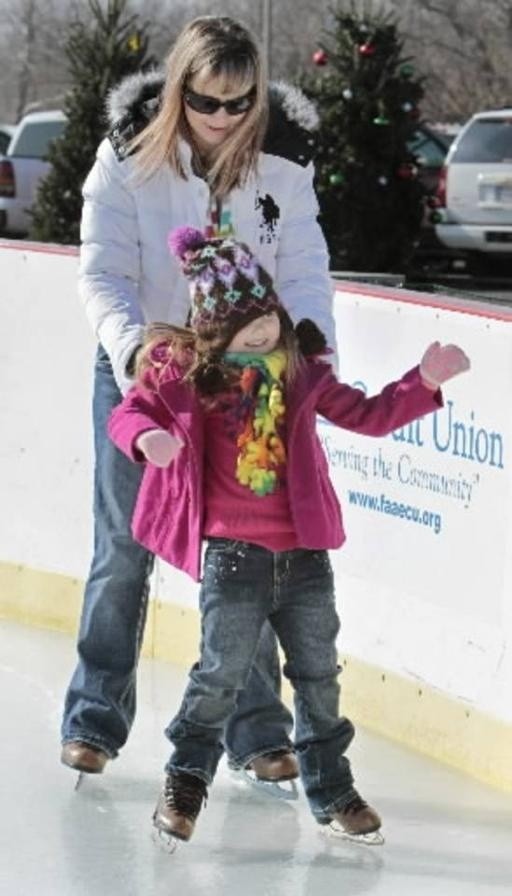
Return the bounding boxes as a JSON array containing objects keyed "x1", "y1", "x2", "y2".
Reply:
[
  {"x1": 0, "y1": 108, "x2": 70, "y2": 239},
  {"x1": 403, "y1": 109, "x2": 512, "y2": 276}
]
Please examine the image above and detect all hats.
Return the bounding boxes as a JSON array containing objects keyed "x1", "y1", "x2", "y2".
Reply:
[{"x1": 169, "y1": 227, "x2": 295, "y2": 390}]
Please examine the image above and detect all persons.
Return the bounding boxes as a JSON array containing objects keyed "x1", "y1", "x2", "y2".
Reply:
[
  {"x1": 61, "y1": 16, "x2": 338, "y2": 783},
  {"x1": 106, "y1": 226, "x2": 471, "y2": 842}
]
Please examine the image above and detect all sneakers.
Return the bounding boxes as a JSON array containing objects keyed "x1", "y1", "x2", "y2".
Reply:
[
  {"x1": 61, "y1": 740, "x2": 106, "y2": 772},
  {"x1": 315, "y1": 797, "x2": 380, "y2": 835},
  {"x1": 244, "y1": 751, "x2": 299, "y2": 782},
  {"x1": 154, "y1": 772, "x2": 205, "y2": 840}
]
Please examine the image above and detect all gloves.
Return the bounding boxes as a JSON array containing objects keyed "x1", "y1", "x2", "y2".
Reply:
[
  {"x1": 135, "y1": 428, "x2": 183, "y2": 466},
  {"x1": 418, "y1": 341, "x2": 470, "y2": 393},
  {"x1": 297, "y1": 319, "x2": 326, "y2": 357}
]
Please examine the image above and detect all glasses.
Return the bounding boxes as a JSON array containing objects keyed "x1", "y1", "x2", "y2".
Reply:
[{"x1": 182, "y1": 81, "x2": 255, "y2": 115}]
